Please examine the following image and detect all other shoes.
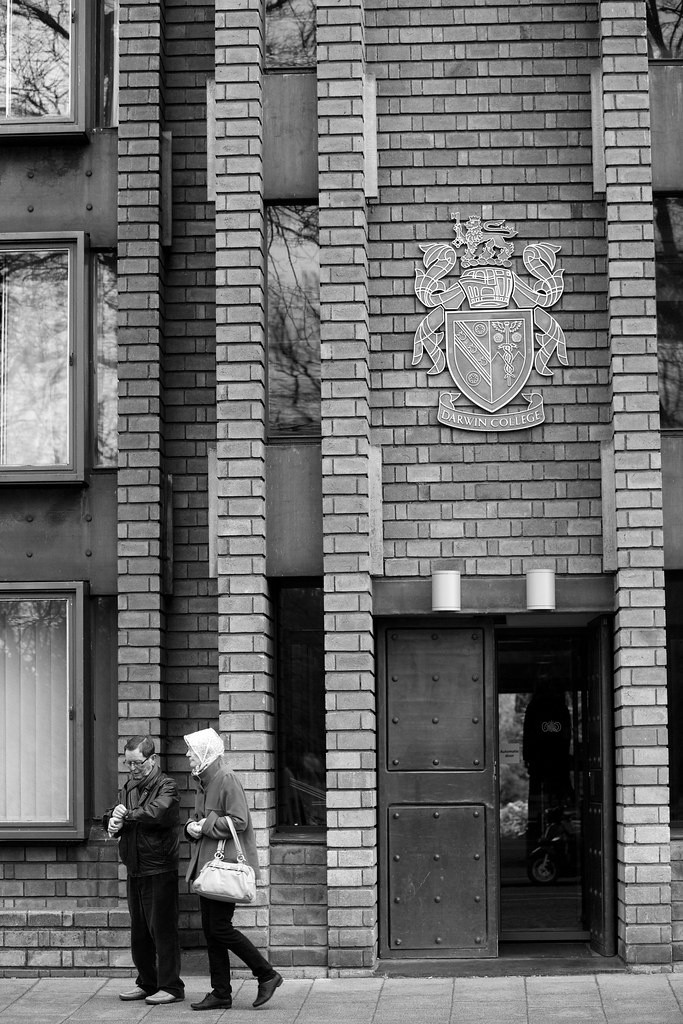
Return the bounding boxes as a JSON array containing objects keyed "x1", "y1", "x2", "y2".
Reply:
[
  {"x1": 191, "y1": 994, "x2": 232, "y2": 1011},
  {"x1": 120, "y1": 985, "x2": 148, "y2": 1000},
  {"x1": 144, "y1": 989, "x2": 185, "y2": 1005},
  {"x1": 252, "y1": 972, "x2": 284, "y2": 1008}
]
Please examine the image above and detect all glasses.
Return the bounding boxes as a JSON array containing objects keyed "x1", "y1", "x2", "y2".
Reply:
[{"x1": 123, "y1": 756, "x2": 149, "y2": 766}]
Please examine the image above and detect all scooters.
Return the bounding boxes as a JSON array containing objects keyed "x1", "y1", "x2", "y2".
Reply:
[{"x1": 527, "y1": 802, "x2": 582, "y2": 885}]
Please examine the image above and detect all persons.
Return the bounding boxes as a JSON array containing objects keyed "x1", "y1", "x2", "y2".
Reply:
[
  {"x1": 102, "y1": 736, "x2": 186, "y2": 1004},
  {"x1": 183, "y1": 728, "x2": 283, "y2": 1010}
]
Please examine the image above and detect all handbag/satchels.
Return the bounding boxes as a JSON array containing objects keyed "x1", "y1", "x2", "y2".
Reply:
[{"x1": 193, "y1": 816, "x2": 256, "y2": 903}]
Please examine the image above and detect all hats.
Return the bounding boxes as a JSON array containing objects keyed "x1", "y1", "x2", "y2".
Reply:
[{"x1": 184, "y1": 727, "x2": 225, "y2": 766}]
